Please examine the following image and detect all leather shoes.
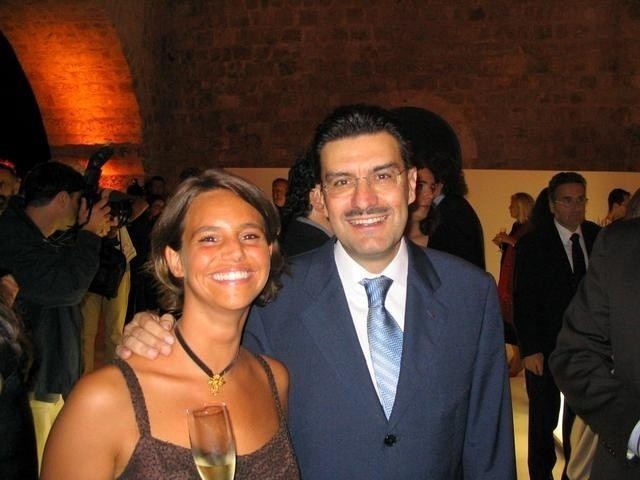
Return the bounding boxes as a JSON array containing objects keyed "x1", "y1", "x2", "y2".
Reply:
[{"x1": 509, "y1": 366, "x2": 522, "y2": 377}]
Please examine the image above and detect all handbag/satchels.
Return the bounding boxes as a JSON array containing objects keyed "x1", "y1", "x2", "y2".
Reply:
[{"x1": 91, "y1": 242, "x2": 127, "y2": 299}]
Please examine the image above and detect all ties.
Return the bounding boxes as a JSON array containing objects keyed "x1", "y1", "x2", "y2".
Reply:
[
  {"x1": 361, "y1": 277, "x2": 403, "y2": 423},
  {"x1": 570, "y1": 233, "x2": 587, "y2": 275}
]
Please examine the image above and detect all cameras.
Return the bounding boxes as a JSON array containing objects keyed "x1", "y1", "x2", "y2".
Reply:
[{"x1": 79, "y1": 188, "x2": 132, "y2": 229}]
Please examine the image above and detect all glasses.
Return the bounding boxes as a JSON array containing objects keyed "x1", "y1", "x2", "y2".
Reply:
[
  {"x1": 553, "y1": 196, "x2": 588, "y2": 208},
  {"x1": 322, "y1": 168, "x2": 408, "y2": 196}
]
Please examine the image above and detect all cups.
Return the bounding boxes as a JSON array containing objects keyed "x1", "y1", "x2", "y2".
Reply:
[{"x1": 187, "y1": 400, "x2": 237, "y2": 479}]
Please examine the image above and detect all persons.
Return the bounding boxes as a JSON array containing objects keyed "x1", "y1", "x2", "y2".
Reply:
[
  {"x1": 1, "y1": 102, "x2": 517, "y2": 479},
  {"x1": 491, "y1": 172, "x2": 639, "y2": 480}
]
